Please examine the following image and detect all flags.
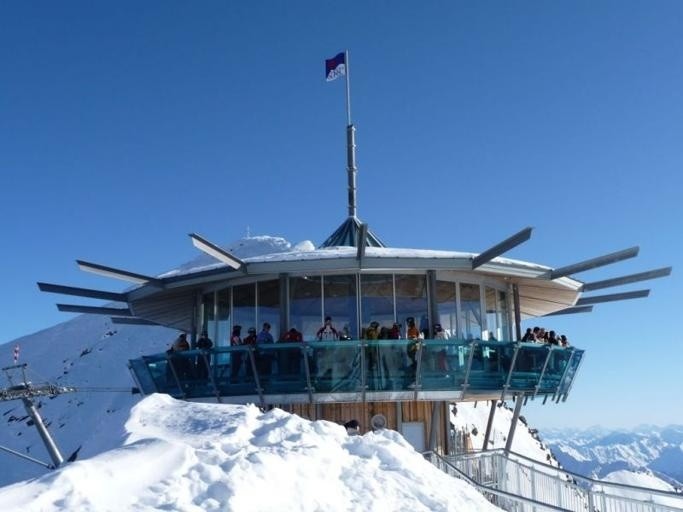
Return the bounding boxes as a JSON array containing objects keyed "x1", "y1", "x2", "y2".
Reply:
[{"x1": 326, "y1": 53, "x2": 346, "y2": 84}]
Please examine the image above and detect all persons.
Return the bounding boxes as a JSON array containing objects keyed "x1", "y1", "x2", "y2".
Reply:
[
  {"x1": 521, "y1": 326, "x2": 568, "y2": 374},
  {"x1": 170, "y1": 333, "x2": 189, "y2": 383},
  {"x1": 231, "y1": 323, "x2": 303, "y2": 384},
  {"x1": 433, "y1": 324, "x2": 449, "y2": 370},
  {"x1": 316, "y1": 316, "x2": 421, "y2": 389},
  {"x1": 197, "y1": 330, "x2": 213, "y2": 384}
]
